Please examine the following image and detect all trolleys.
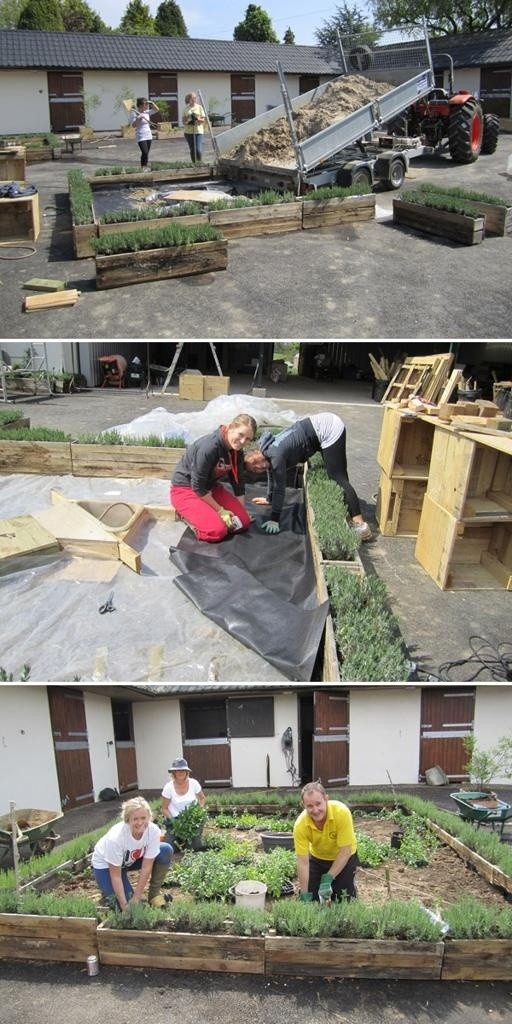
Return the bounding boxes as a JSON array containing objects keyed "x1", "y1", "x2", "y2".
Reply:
[
  {"x1": 0, "y1": 808, "x2": 64, "y2": 863},
  {"x1": 449, "y1": 791, "x2": 512, "y2": 836}
]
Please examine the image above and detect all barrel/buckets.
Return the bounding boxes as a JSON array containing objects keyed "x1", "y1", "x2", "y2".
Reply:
[
  {"x1": 390, "y1": 830, "x2": 404, "y2": 849},
  {"x1": 229, "y1": 880, "x2": 267, "y2": 911}
]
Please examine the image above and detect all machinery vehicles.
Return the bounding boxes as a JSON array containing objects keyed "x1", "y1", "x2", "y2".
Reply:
[{"x1": 197, "y1": 13, "x2": 500, "y2": 195}]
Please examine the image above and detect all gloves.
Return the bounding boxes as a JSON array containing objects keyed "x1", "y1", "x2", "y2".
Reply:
[
  {"x1": 252, "y1": 497, "x2": 269, "y2": 506},
  {"x1": 261, "y1": 519, "x2": 280, "y2": 535},
  {"x1": 299, "y1": 891, "x2": 312, "y2": 906},
  {"x1": 215, "y1": 506, "x2": 237, "y2": 529},
  {"x1": 318, "y1": 872, "x2": 333, "y2": 905}
]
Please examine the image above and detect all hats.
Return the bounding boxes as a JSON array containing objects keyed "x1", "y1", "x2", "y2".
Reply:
[{"x1": 167, "y1": 756, "x2": 192, "y2": 776}]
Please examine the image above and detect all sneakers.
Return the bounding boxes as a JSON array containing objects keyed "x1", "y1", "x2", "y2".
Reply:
[{"x1": 353, "y1": 523, "x2": 373, "y2": 542}]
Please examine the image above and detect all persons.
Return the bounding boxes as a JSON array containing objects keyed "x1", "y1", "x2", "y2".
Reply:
[
  {"x1": 162, "y1": 757, "x2": 205, "y2": 853},
  {"x1": 181, "y1": 92, "x2": 207, "y2": 164},
  {"x1": 130, "y1": 97, "x2": 160, "y2": 168},
  {"x1": 91, "y1": 796, "x2": 174, "y2": 911},
  {"x1": 168, "y1": 413, "x2": 257, "y2": 543},
  {"x1": 243, "y1": 412, "x2": 373, "y2": 542},
  {"x1": 292, "y1": 782, "x2": 358, "y2": 904}
]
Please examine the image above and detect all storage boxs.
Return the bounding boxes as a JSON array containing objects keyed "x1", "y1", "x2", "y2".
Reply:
[
  {"x1": 427, "y1": 425, "x2": 512, "y2": 523},
  {"x1": 0, "y1": 193, "x2": 40, "y2": 247},
  {"x1": 414, "y1": 493, "x2": 512, "y2": 591},
  {"x1": 203, "y1": 376, "x2": 230, "y2": 401},
  {"x1": 376, "y1": 406, "x2": 434, "y2": 480},
  {"x1": 178, "y1": 374, "x2": 203, "y2": 401},
  {"x1": 374, "y1": 468, "x2": 428, "y2": 538}
]
